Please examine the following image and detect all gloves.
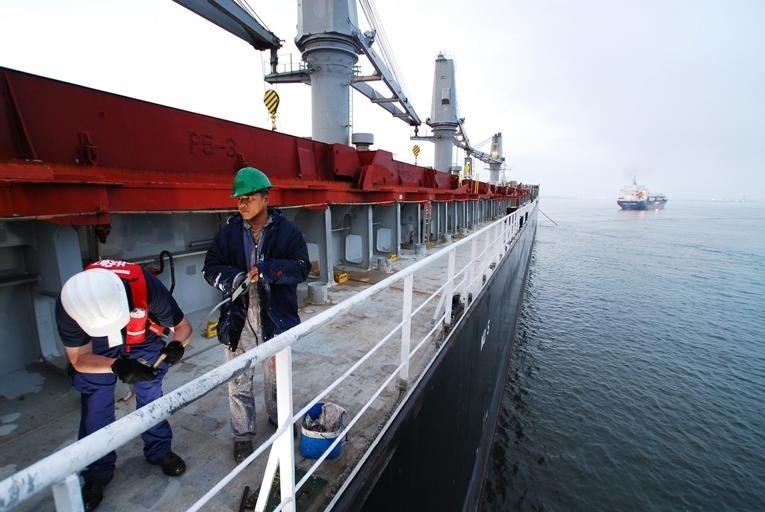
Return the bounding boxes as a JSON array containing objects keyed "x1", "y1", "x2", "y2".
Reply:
[
  {"x1": 110, "y1": 358, "x2": 161, "y2": 385},
  {"x1": 159, "y1": 340, "x2": 185, "y2": 365}
]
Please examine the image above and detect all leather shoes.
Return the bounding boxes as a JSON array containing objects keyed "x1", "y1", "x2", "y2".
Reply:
[
  {"x1": 82, "y1": 472, "x2": 115, "y2": 512},
  {"x1": 145, "y1": 450, "x2": 186, "y2": 476},
  {"x1": 234, "y1": 440, "x2": 254, "y2": 465},
  {"x1": 268, "y1": 416, "x2": 297, "y2": 439}
]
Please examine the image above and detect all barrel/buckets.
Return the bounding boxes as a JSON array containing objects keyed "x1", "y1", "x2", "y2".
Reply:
[{"x1": 299, "y1": 413, "x2": 341, "y2": 460}]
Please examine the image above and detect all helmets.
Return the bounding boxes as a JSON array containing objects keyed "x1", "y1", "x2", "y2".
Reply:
[
  {"x1": 60, "y1": 266, "x2": 132, "y2": 348},
  {"x1": 229, "y1": 167, "x2": 272, "y2": 198}
]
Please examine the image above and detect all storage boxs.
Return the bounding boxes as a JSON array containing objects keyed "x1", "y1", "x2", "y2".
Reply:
[{"x1": 300, "y1": 402, "x2": 344, "y2": 458}]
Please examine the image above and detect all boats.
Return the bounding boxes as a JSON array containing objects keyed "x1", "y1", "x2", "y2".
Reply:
[{"x1": 614, "y1": 176, "x2": 670, "y2": 211}]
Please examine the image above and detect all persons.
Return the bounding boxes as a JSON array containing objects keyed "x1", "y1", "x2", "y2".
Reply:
[
  {"x1": 54, "y1": 257, "x2": 197, "y2": 511},
  {"x1": 199, "y1": 166, "x2": 313, "y2": 466}
]
